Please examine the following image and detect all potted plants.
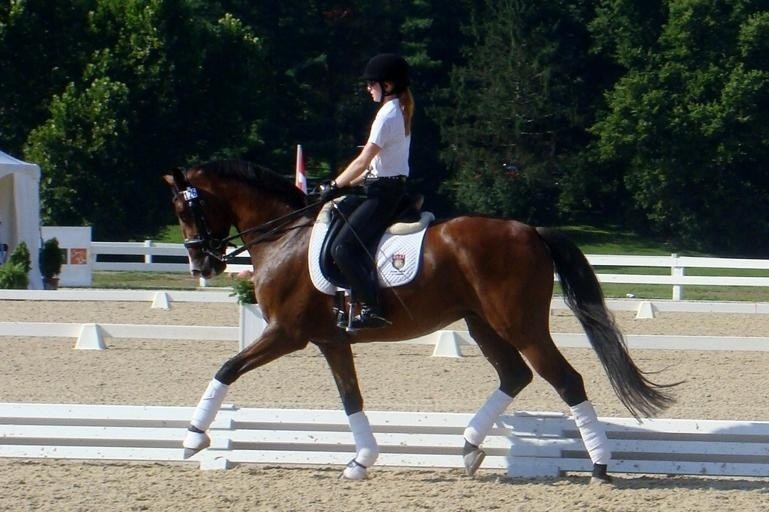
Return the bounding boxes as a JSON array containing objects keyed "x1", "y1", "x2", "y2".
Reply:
[{"x1": 38, "y1": 237, "x2": 64, "y2": 291}]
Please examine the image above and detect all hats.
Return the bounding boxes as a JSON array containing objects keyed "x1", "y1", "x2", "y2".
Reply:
[{"x1": 350, "y1": 53, "x2": 411, "y2": 82}]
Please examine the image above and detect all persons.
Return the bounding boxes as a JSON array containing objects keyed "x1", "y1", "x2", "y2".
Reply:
[{"x1": 315, "y1": 52, "x2": 416, "y2": 332}]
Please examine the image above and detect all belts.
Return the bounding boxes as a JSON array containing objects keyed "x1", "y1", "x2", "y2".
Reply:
[{"x1": 382, "y1": 176, "x2": 406, "y2": 182}]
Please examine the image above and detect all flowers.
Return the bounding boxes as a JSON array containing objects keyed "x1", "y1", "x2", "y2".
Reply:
[{"x1": 227, "y1": 267, "x2": 256, "y2": 303}]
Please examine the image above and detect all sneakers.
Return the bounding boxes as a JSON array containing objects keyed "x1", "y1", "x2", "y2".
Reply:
[{"x1": 335, "y1": 308, "x2": 393, "y2": 331}]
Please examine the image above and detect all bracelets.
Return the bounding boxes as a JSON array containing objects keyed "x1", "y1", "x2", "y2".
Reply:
[{"x1": 329, "y1": 177, "x2": 339, "y2": 190}]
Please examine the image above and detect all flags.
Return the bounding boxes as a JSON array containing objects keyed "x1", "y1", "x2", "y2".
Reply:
[{"x1": 295, "y1": 144, "x2": 310, "y2": 196}]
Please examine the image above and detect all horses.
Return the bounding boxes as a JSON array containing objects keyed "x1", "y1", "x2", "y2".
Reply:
[{"x1": 162, "y1": 157, "x2": 690, "y2": 490}]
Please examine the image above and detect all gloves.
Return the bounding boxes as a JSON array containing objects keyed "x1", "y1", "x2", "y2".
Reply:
[{"x1": 319, "y1": 180, "x2": 339, "y2": 198}]
